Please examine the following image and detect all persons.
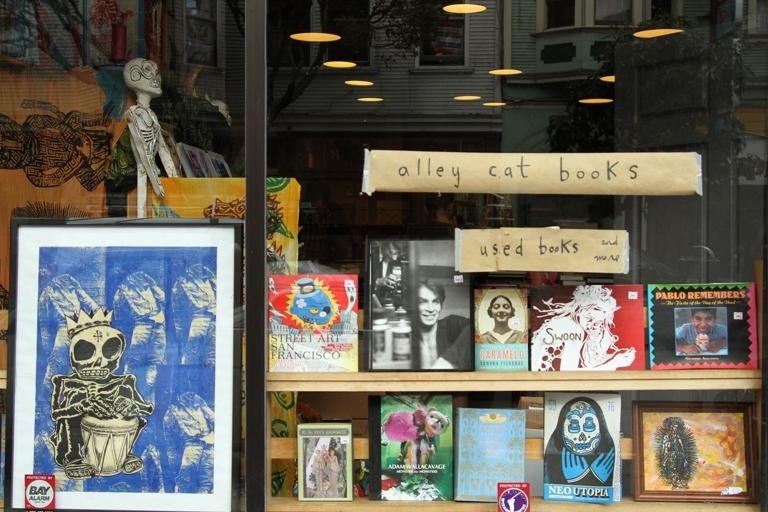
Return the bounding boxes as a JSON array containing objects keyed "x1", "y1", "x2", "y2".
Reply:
[
  {"x1": 475, "y1": 294, "x2": 527, "y2": 344},
  {"x1": 373, "y1": 241, "x2": 403, "y2": 306},
  {"x1": 675, "y1": 308, "x2": 728, "y2": 355},
  {"x1": 418, "y1": 279, "x2": 474, "y2": 370}
]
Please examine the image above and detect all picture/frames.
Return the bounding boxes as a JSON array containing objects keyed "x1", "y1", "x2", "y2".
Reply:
[
  {"x1": 160, "y1": 129, "x2": 232, "y2": 178},
  {"x1": 5, "y1": 216, "x2": 244, "y2": 512},
  {"x1": 632, "y1": 400, "x2": 760, "y2": 504}
]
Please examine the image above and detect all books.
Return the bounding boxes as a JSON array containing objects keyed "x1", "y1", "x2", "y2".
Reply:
[
  {"x1": 647, "y1": 284, "x2": 758, "y2": 368},
  {"x1": 267, "y1": 272, "x2": 360, "y2": 374},
  {"x1": 530, "y1": 285, "x2": 645, "y2": 369},
  {"x1": 297, "y1": 423, "x2": 353, "y2": 501},
  {"x1": 543, "y1": 391, "x2": 623, "y2": 503},
  {"x1": 381, "y1": 395, "x2": 453, "y2": 500},
  {"x1": 475, "y1": 288, "x2": 530, "y2": 372},
  {"x1": 454, "y1": 409, "x2": 526, "y2": 502}
]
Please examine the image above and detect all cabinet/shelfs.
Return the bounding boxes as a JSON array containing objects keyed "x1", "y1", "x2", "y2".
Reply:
[{"x1": 264, "y1": 370, "x2": 763, "y2": 512}]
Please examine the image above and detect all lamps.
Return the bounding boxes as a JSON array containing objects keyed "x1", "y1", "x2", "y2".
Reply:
[
  {"x1": 323, "y1": 42, "x2": 360, "y2": 69},
  {"x1": 357, "y1": 84, "x2": 385, "y2": 102},
  {"x1": 344, "y1": 69, "x2": 376, "y2": 86},
  {"x1": 291, "y1": 4, "x2": 343, "y2": 42},
  {"x1": 484, "y1": 101, "x2": 506, "y2": 105},
  {"x1": 489, "y1": 63, "x2": 522, "y2": 74},
  {"x1": 577, "y1": 87, "x2": 614, "y2": 103},
  {"x1": 596, "y1": 60, "x2": 616, "y2": 84},
  {"x1": 456, "y1": 94, "x2": 481, "y2": 100},
  {"x1": 633, "y1": 21, "x2": 682, "y2": 40},
  {"x1": 442, "y1": 2, "x2": 487, "y2": 14}
]
